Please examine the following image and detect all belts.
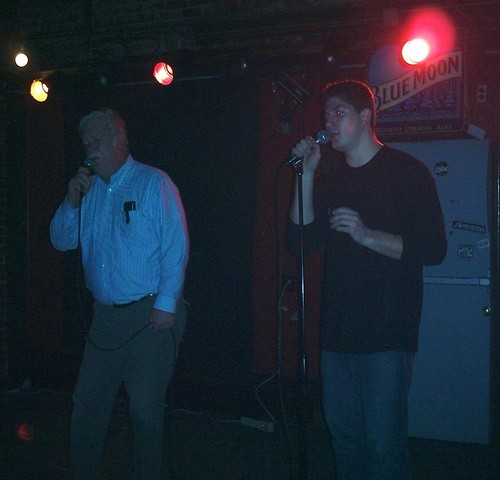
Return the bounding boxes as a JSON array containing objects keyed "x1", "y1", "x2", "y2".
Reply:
[{"x1": 111, "y1": 293, "x2": 155, "y2": 310}]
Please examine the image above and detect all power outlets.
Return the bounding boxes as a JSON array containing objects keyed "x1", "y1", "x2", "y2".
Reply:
[
  {"x1": 477, "y1": 84, "x2": 487, "y2": 103},
  {"x1": 281, "y1": 275, "x2": 295, "y2": 293}
]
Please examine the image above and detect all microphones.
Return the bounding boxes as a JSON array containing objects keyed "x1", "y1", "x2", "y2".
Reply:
[
  {"x1": 279, "y1": 129, "x2": 330, "y2": 168},
  {"x1": 79, "y1": 156, "x2": 96, "y2": 196}
]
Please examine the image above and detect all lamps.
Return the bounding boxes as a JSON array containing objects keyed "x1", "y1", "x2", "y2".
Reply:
[
  {"x1": 154, "y1": 48, "x2": 194, "y2": 86},
  {"x1": 30, "y1": 71, "x2": 65, "y2": 102}
]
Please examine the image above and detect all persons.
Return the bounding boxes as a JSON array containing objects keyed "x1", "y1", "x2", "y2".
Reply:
[
  {"x1": 49, "y1": 110, "x2": 191, "y2": 480},
  {"x1": 285, "y1": 81, "x2": 448, "y2": 480}
]
